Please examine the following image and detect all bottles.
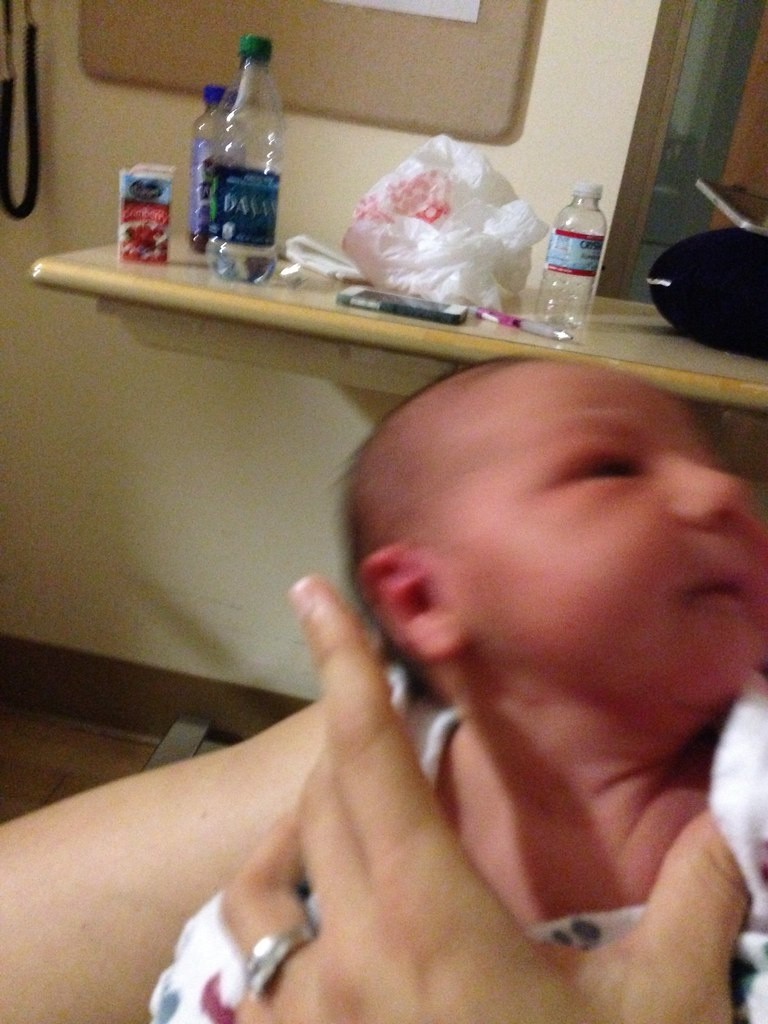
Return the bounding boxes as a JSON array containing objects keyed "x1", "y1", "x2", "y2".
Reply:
[
  {"x1": 186, "y1": 84, "x2": 228, "y2": 252},
  {"x1": 204, "y1": 34, "x2": 284, "y2": 285},
  {"x1": 532, "y1": 181, "x2": 607, "y2": 330}
]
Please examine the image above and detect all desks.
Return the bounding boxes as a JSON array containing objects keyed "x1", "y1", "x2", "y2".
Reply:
[{"x1": 30, "y1": 231, "x2": 767, "y2": 414}]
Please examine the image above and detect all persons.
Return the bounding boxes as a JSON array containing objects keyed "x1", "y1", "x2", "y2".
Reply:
[
  {"x1": 0, "y1": 659, "x2": 768, "y2": 1024},
  {"x1": 146, "y1": 357, "x2": 767, "y2": 1024}
]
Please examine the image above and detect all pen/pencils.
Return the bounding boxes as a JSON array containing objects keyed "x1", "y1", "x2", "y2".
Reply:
[{"x1": 468, "y1": 305, "x2": 576, "y2": 341}]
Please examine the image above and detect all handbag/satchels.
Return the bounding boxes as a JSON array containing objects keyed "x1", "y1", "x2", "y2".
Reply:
[{"x1": 342, "y1": 133, "x2": 547, "y2": 311}]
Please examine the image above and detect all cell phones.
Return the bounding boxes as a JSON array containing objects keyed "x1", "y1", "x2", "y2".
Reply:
[
  {"x1": 696, "y1": 178, "x2": 768, "y2": 237},
  {"x1": 337, "y1": 286, "x2": 469, "y2": 324}
]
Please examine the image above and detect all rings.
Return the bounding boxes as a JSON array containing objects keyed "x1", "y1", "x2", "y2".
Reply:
[{"x1": 243, "y1": 926, "x2": 316, "y2": 996}]
